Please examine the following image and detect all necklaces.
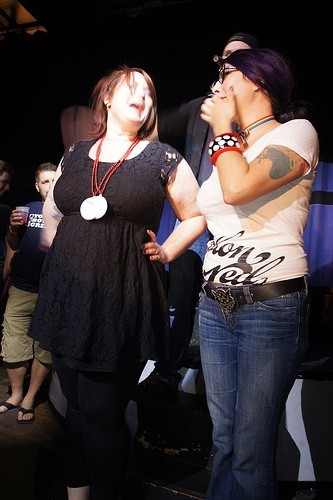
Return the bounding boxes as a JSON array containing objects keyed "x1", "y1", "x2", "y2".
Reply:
[
  {"x1": 234, "y1": 114, "x2": 276, "y2": 145},
  {"x1": 80, "y1": 134, "x2": 138, "y2": 221},
  {"x1": 95, "y1": 137, "x2": 141, "y2": 219}
]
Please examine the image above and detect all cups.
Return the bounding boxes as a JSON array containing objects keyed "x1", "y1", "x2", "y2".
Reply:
[{"x1": 16, "y1": 207, "x2": 30, "y2": 224}]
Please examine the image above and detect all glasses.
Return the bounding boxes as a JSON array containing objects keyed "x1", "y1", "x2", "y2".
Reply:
[{"x1": 210, "y1": 64, "x2": 238, "y2": 90}]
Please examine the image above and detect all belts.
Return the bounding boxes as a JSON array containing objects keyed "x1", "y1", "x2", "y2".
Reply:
[{"x1": 200, "y1": 276, "x2": 307, "y2": 313}]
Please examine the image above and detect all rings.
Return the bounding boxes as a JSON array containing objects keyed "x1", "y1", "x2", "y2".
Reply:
[{"x1": 156, "y1": 249, "x2": 159, "y2": 254}]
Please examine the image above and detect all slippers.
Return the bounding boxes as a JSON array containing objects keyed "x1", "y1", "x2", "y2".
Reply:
[
  {"x1": 0, "y1": 399, "x2": 21, "y2": 415},
  {"x1": 16, "y1": 406, "x2": 36, "y2": 425}
]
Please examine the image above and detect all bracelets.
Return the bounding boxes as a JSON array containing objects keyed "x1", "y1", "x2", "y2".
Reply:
[{"x1": 207, "y1": 131, "x2": 243, "y2": 164}]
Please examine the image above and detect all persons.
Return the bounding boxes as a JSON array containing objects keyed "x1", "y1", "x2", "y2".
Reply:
[{"x1": 0, "y1": 30, "x2": 319, "y2": 500}]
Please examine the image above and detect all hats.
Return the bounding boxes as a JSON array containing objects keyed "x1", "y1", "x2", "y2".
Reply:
[{"x1": 226, "y1": 32, "x2": 257, "y2": 50}]
